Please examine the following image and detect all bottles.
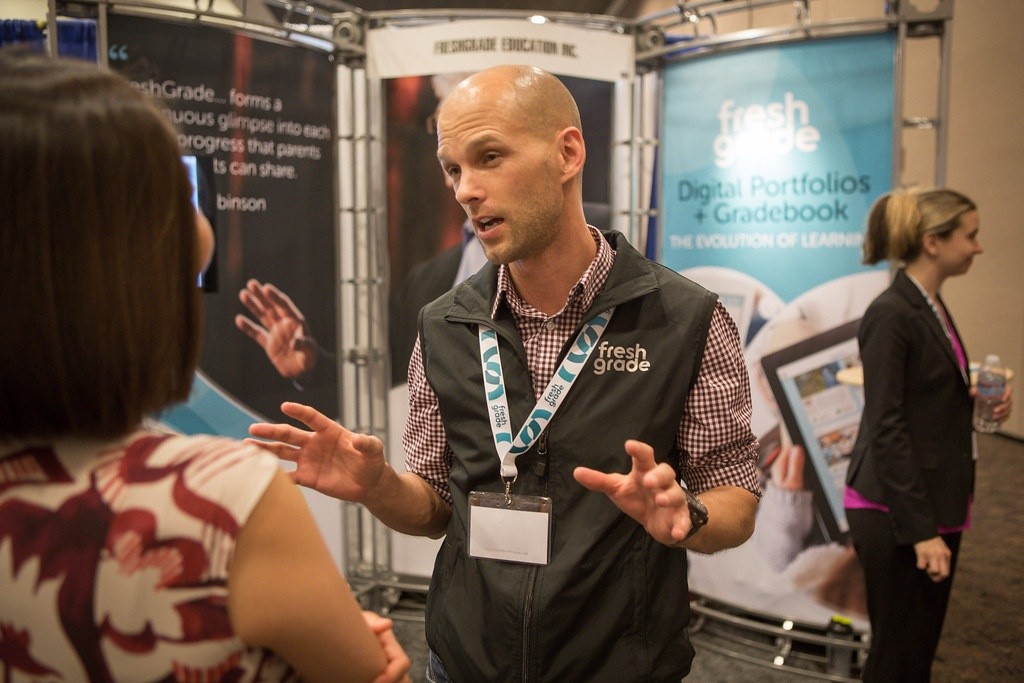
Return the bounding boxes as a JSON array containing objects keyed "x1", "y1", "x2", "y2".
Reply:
[{"x1": 975, "y1": 355, "x2": 1005, "y2": 434}]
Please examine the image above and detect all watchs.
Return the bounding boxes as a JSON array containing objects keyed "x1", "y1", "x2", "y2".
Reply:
[{"x1": 682, "y1": 486, "x2": 709, "y2": 539}]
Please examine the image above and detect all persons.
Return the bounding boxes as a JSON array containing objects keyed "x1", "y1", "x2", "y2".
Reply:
[
  {"x1": 843, "y1": 190, "x2": 1015, "y2": 683},
  {"x1": 238, "y1": 65, "x2": 765, "y2": 683},
  {"x1": 0, "y1": 46, "x2": 410, "y2": 683},
  {"x1": 234, "y1": 218, "x2": 863, "y2": 626}
]
